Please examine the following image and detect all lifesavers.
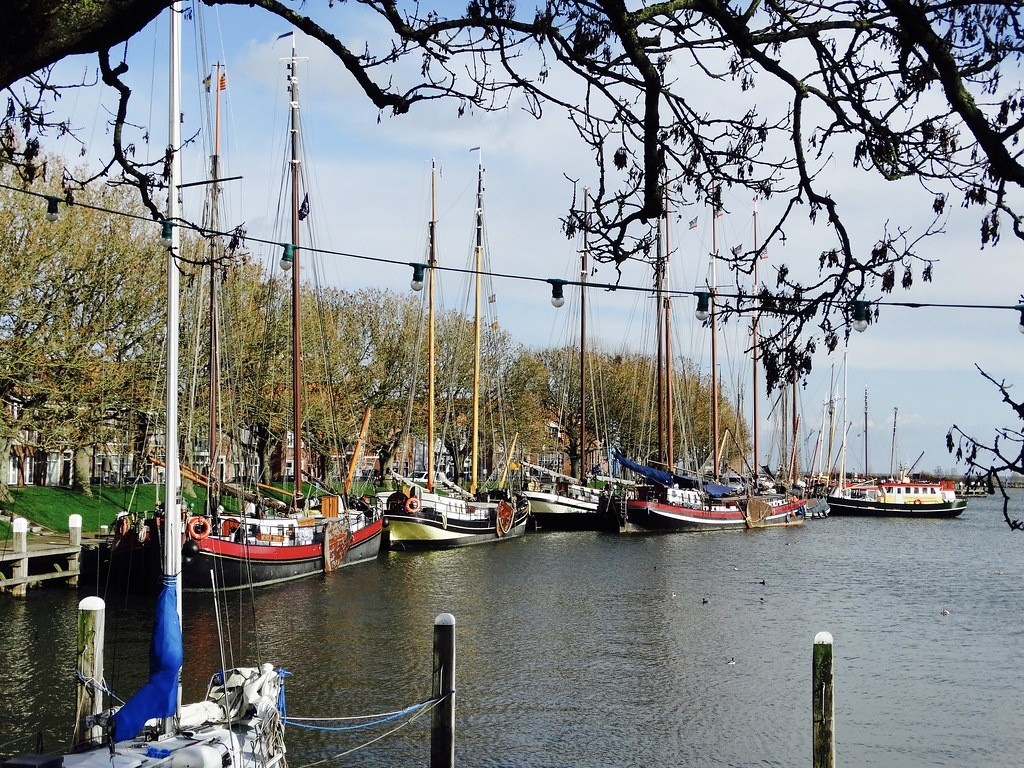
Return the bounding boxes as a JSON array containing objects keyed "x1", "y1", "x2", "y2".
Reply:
[
  {"x1": 190, "y1": 517, "x2": 212, "y2": 540},
  {"x1": 406, "y1": 497, "x2": 420, "y2": 513},
  {"x1": 914, "y1": 499, "x2": 921, "y2": 504}
]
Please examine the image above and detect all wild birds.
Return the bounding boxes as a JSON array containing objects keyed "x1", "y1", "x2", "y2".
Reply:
[
  {"x1": 759, "y1": 580, "x2": 766, "y2": 586},
  {"x1": 734, "y1": 565, "x2": 740, "y2": 571},
  {"x1": 671, "y1": 590, "x2": 677, "y2": 597},
  {"x1": 725, "y1": 657, "x2": 736, "y2": 665},
  {"x1": 998, "y1": 568, "x2": 1006, "y2": 575},
  {"x1": 794, "y1": 540, "x2": 800, "y2": 544},
  {"x1": 702, "y1": 598, "x2": 708, "y2": 605},
  {"x1": 760, "y1": 598, "x2": 769, "y2": 605},
  {"x1": 785, "y1": 541, "x2": 792, "y2": 545},
  {"x1": 941, "y1": 607, "x2": 951, "y2": 616}
]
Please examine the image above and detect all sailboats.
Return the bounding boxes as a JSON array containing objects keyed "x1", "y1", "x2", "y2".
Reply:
[
  {"x1": 0, "y1": 0, "x2": 995, "y2": 589},
  {"x1": 44, "y1": 0, "x2": 293, "y2": 768}
]
